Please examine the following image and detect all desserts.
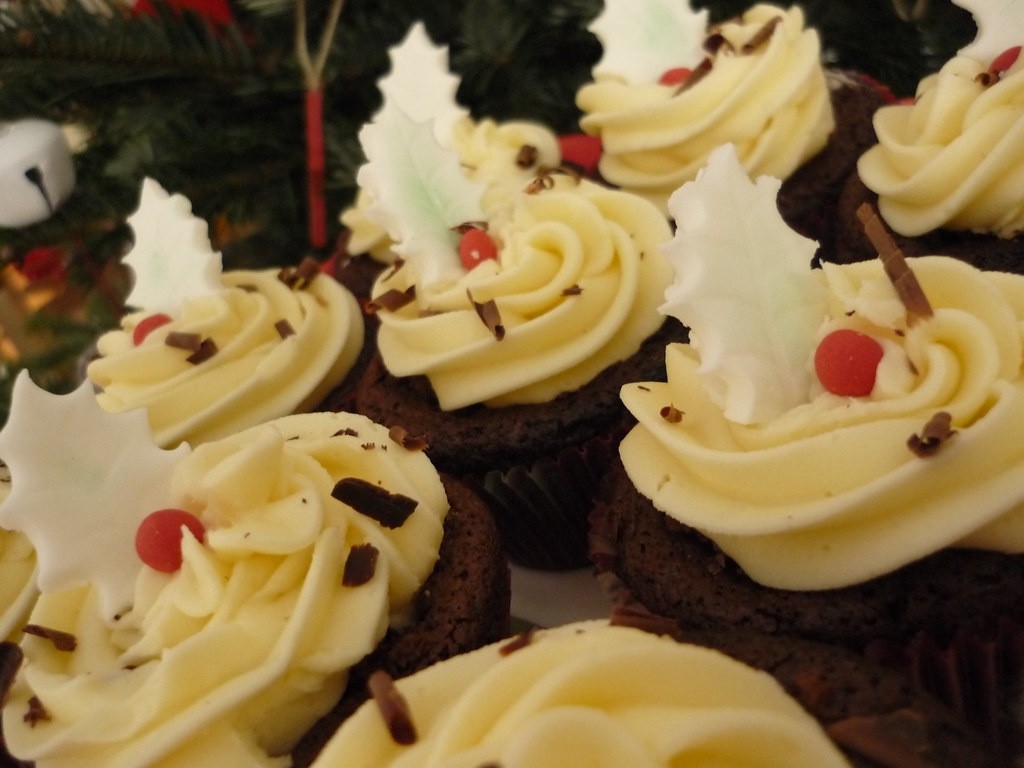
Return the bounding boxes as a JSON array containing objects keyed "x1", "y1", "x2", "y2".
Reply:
[{"x1": 0, "y1": 0, "x2": 1024, "y2": 768}]
